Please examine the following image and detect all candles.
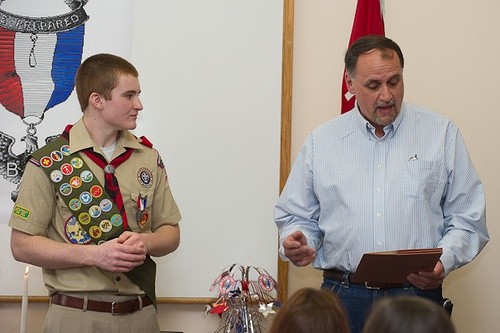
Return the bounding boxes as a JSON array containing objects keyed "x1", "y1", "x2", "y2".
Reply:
[{"x1": 19, "y1": 266, "x2": 29, "y2": 333}]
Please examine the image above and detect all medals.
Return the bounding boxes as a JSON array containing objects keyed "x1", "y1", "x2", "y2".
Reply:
[{"x1": 136, "y1": 209, "x2": 149, "y2": 230}]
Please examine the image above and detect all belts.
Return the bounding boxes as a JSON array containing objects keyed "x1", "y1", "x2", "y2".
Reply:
[
  {"x1": 323, "y1": 271, "x2": 405, "y2": 290},
  {"x1": 51, "y1": 292, "x2": 153, "y2": 315}
]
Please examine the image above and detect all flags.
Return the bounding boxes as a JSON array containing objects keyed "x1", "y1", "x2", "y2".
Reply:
[{"x1": 341, "y1": 0, "x2": 385, "y2": 115}]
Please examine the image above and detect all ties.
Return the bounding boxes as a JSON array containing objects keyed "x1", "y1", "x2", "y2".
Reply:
[{"x1": 62, "y1": 124, "x2": 154, "y2": 230}]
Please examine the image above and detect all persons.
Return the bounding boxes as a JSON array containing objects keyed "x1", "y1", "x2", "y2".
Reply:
[
  {"x1": 274, "y1": 33, "x2": 490, "y2": 333},
  {"x1": 270, "y1": 287, "x2": 352, "y2": 333},
  {"x1": 7, "y1": 52, "x2": 182, "y2": 332},
  {"x1": 363, "y1": 295, "x2": 457, "y2": 333}
]
